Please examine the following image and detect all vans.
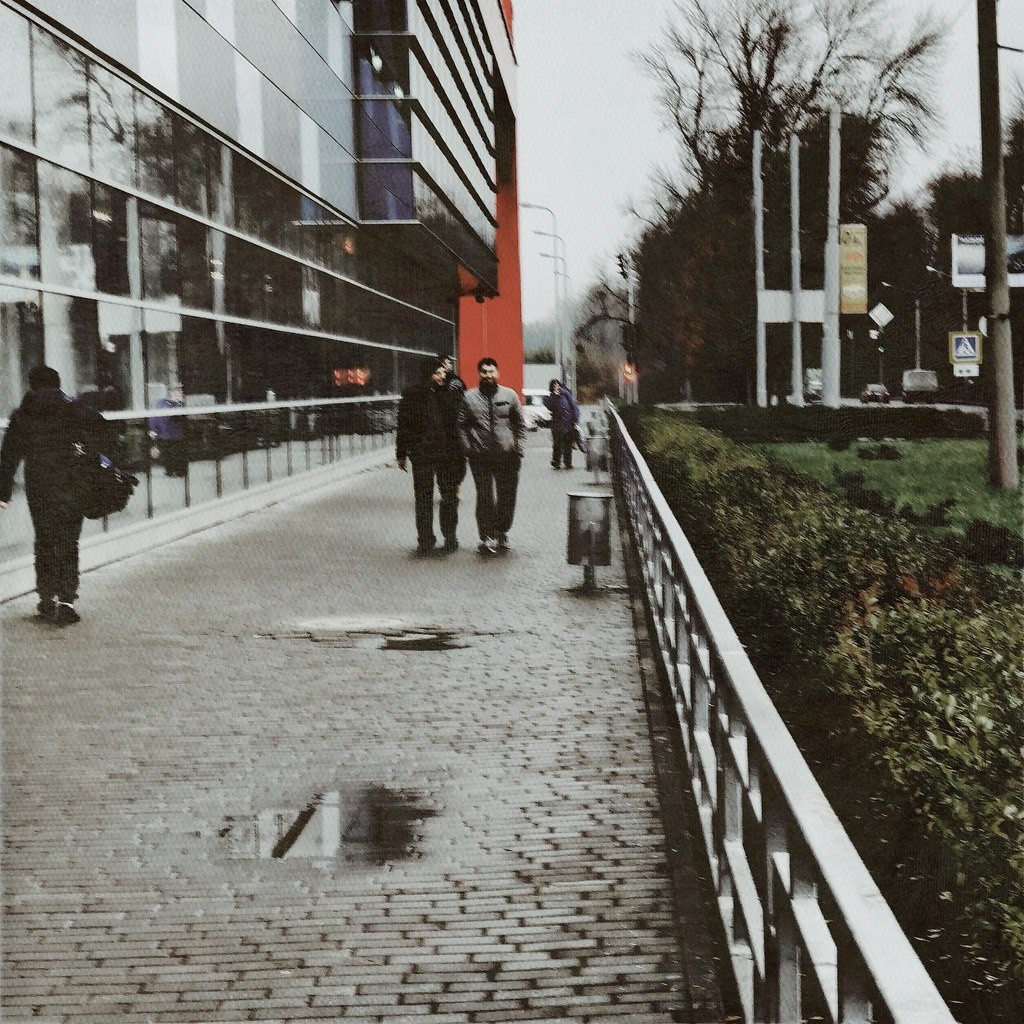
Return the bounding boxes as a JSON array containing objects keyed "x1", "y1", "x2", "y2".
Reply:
[{"x1": 804, "y1": 368, "x2": 823, "y2": 399}]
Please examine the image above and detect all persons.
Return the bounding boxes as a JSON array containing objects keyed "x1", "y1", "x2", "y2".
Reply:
[
  {"x1": 437, "y1": 354, "x2": 467, "y2": 497},
  {"x1": 0, "y1": 365, "x2": 112, "y2": 620},
  {"x1": 548, "y1": 379, "x2": 580, "y2": 469},
  {"x1": 455, "y1": 358, "x2": 528, "y2": 554},
  {"x1": 396, "y1": 360, "x2": 463, "y2": 556}
]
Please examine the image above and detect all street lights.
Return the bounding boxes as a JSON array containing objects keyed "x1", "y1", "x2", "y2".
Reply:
[
  {"x1": 521, "y1": 202, "x2": 572, "y2": 390},
  {"x1": 882, "y1": 281, "x2": 922, "y2": 369},
  {"x1": 925, "y1": 265, "x2": 967, "y2": 401}
]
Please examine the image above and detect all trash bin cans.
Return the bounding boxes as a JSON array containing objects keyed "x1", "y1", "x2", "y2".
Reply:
[
  {"x1": 584, "y1": 436, "x2": 608, "y2": 486},
  {"x1": 565, "y1": 492, "x2": 614, "y2": 592}
]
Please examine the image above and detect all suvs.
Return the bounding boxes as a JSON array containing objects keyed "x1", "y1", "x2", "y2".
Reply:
[{"x1": 860, "y1": 384, "x2": 890, "y2": 404}]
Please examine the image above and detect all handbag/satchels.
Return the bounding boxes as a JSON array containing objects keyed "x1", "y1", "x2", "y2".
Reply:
[
  {"x1": 62, "y1": 442, "x2": 138, "y2": 519},
  {"x1": 573, "y1": 422, "x2": 587, "y2": 453}
]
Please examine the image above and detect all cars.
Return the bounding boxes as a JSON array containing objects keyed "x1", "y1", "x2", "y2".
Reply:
[
  {"x1": 523, "y1": 389, "x2": 552, "y2": 424},
  {"x1": 117, "y1": 416, "x2": 241, "y2": 465}
]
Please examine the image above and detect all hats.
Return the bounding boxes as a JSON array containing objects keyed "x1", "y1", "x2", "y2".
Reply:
[
  {"x1": 28, "y1": 365, "x2": 61, "y2": 392},
  {"x1": 420, "y1": 357, "x2": 441, "y2": 378}
]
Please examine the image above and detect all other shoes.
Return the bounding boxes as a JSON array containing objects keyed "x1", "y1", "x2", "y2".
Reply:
[
  {"x1": 36, "y1": 598, "x2": 58, "y2": 620},
  {"x1": 550, "y1": 460, "x2": 560, "y2": 469},
  {"x1": 444, "y1": 539, "x2": 460, "y2": 554},
  {"x1": 56, "y1": 602, "x2": 80, "y2": 627},
  {"x1": 417, "y1": 541, "x2": 435, "y2": 557},
  {"x1": 496, "y1": 536, "x2": 512, "y2": 550},
  {"x1": 565, "y1": 463, "x2": 574, "y2": 469},
  {"x1": 477, "y1": 536, "x2": 497, "y2": 556}
]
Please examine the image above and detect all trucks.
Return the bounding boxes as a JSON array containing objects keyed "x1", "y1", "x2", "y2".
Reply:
[{"x1": 901, "y1": 367, "x2": 938, "y2": 404}]
